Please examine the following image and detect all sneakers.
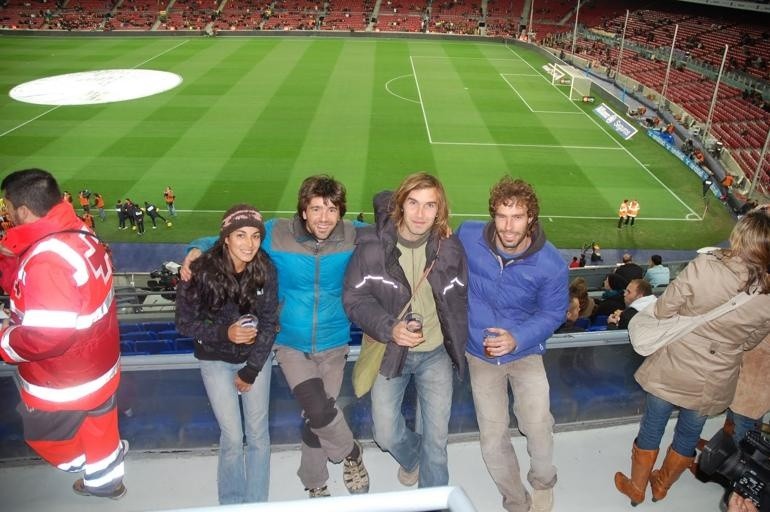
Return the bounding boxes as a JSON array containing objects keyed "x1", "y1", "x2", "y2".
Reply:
[
  {"x1": 397, "y1": 466, "x2": 420, "y2": 487},
  {"x1": 532, "y1": 485, "x2": 554, "y2": 512},
  {"x1": 73, "y1": 478, "x2": 127, "y2": 500}
]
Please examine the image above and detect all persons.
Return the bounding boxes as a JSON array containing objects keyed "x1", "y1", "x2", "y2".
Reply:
[
  {"x1": 174, "y1": 171, "x2": 568, "y2": 512},
  {"x1": 614, "y1": 199, "x2": 770, "y2": 510},
  {"x1": 535, "y1": 8, "x2": 770, "y2": 214},
  {"x1": 0, "y1": 169, "x2": 129, "y2": 500},
  {"x1": 553, "y1": 243, "x2": 669, "y2": 334},
  {"x1": 0, "y1": 1, "x2": 529, "y2": 42},
  {"x1": 63, "y1": 187, "x2": 176, "y2": 236},
  {"x1": 0, "y1": 199, "x2": 16, "y2": 242}
]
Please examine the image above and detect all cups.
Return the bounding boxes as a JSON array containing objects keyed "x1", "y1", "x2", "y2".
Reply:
[
  {"x1": 238, "y1": 314, "x2": 258, "y2": 345},
  {"x1": 404, "y1": 313, "x2": 423, "y2": 346},
  {"x1": 481, "y1": 328, "x2": 499, "y2": 359}
]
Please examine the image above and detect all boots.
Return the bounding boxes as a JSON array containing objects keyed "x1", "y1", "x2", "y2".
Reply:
[
  {"x1": 649, "y1": 444, "x2": 696, "y2": 503},
  {"x1": 614, "y1": 437, "x2": 660, "y2": 506}
]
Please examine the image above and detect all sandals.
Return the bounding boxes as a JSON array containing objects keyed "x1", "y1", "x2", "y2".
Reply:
[
  {"x1": 342, "y1": 440, "x2": 370, "y2": 494},
  {"x1": 310, "y1": 487, "x2": 330, "y2": 496}
]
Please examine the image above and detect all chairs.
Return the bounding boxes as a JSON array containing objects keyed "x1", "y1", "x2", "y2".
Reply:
[
  {"x1": 577, "y1": 314, "x2": 609, "y2": 332},
  {"x1": 657, "y1": 284, "x2": 669, "y2": 288},
  {"x1": 118, "y1": 320, "x2": 197, "y2": 356},
  {"x1": 349, "y1": 325, "x2": 362, "y2": 345}
]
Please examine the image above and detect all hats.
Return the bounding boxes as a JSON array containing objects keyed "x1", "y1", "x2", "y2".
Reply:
[
  {"x1": 609, "y1": 272, "x2": 628, "y2": 290},
  {"x1": 221, "y1": 203, "x2": 265, "y2": 242}
]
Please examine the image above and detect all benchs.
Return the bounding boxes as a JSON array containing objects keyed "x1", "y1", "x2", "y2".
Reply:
[{"x1": 0, "y1": 1, "x2": 769, "y2": 201}]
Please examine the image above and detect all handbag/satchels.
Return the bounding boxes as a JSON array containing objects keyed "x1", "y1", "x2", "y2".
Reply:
[
  {"x1": 352, "y1": 332, "x2": 387, "y2": 399},
  {"x1": 627, "y1": 299, "x2": 700, "y2": 357}
]
